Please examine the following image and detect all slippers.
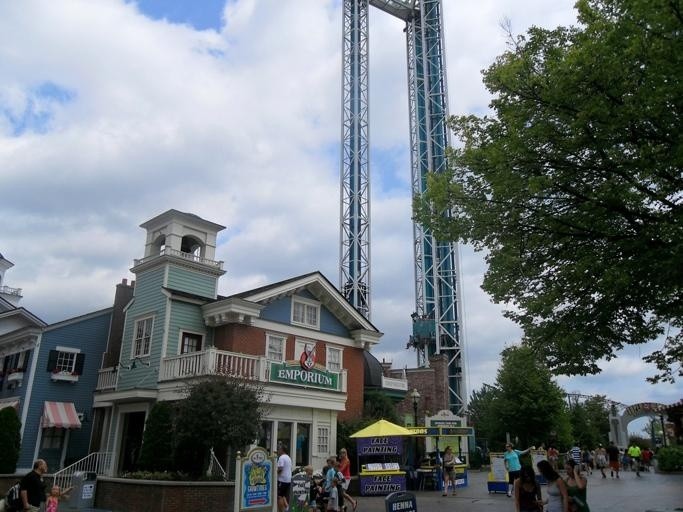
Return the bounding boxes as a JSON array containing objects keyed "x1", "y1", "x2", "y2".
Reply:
[{"x1": 352, "y1": 500, "x2": 357, "y2": 511}]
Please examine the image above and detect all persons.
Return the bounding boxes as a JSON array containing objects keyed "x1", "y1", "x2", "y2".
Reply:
[
  {"x1": 537, "y1": 460, "x2": 568, "y2": 512},
  {"x1": 440, "y1": 445, "x2": 456, "y2": 497},
  {"x1": 538, "y1": 439, "x2": 658, "y2": 480},
  {"x1": 502, "y1": 442, "x2": 537, "y2": 500},
  {"x1": 6, "y1": 482, "x2": 21, "y2": 512},
  {"x1": 275, "y1": 441, "x2": 292, "y2": 512},
  {"x1": 313, "y1": 445, "x2": 358, "y2": 512},
  {"x1": 45, "y1": 485, "x2": 74, "y2": 512},
  {"x1": 563, "y1": 457, "x2": 590, "y2": 512},
  {"x1": 514, "y1": 463, "x2": 545, "y2": 512},
  {"x1": 20, "y1": 458, "x2": 48, "y2": 512}
]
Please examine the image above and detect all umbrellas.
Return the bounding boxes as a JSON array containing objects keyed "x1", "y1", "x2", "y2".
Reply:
[{"x1": 349, "y1": 416, "x2": 415, "y2": 464}]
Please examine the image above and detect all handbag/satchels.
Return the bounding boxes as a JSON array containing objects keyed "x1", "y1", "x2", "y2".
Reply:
[{"x1": 567, "y1": 495, "x2": 584, "y2": 511}]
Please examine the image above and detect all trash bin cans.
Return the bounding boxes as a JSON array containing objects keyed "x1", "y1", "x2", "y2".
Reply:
[{"x1": 69, "y1": 470, "x2": 97, "y2": 509}]
